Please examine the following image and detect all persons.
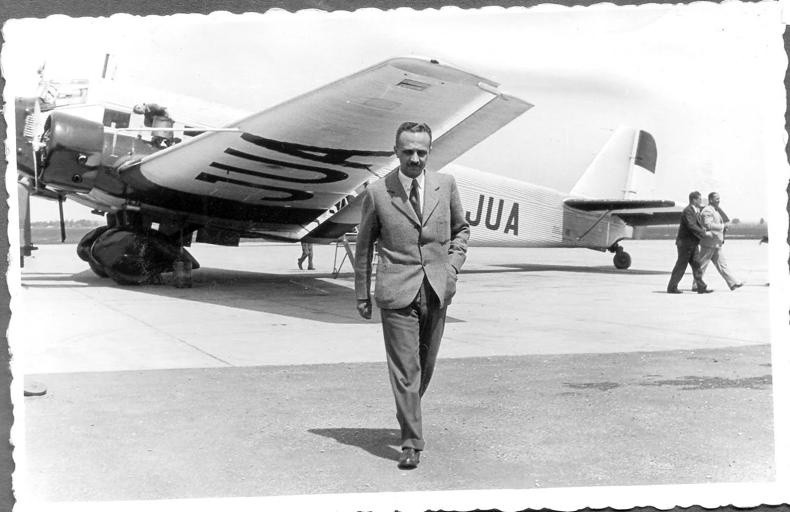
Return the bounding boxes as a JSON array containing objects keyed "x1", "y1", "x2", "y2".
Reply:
[
  {"x1": 297, "y1": 241, "x2": 315, "y2": 270},
  {"x1": 692, "y1": 193, "x2": 745, "y2": 292},
  {"x1": 667, "y1": 192, "x2": 713, "y2": 294},
  {"x1": 354, "y1": 120, "x2": 470, "y2": 469}
]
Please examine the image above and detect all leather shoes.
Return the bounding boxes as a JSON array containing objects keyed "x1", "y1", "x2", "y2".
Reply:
[
  {"x1": 398, "y1": 448, "x2": 421, "y2": 468},
  {"x1": 298, "y1": 263, "x2": 302, "y2": 270},
  {"x1": 667, "y1": 287, "x2": 683, "y2": 293},
  {"x1": 308, "y1": 267, "x2": 315, "y2": 270},
  {"x1": 698, "y1": 288, "x2": 714, "y2": 293},
  {"x1": 730, "y1": 283, "x2": 744, "y2": 290}
]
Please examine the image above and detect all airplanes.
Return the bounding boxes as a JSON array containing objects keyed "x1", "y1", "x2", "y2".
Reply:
[{"x1": 16, "y1": 55, "x2": 686, "y2": 287}]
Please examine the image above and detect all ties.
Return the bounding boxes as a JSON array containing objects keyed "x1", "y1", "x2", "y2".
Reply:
[{"x1": 409, "y1": 179, "x2": 423, "y2": 223}]
